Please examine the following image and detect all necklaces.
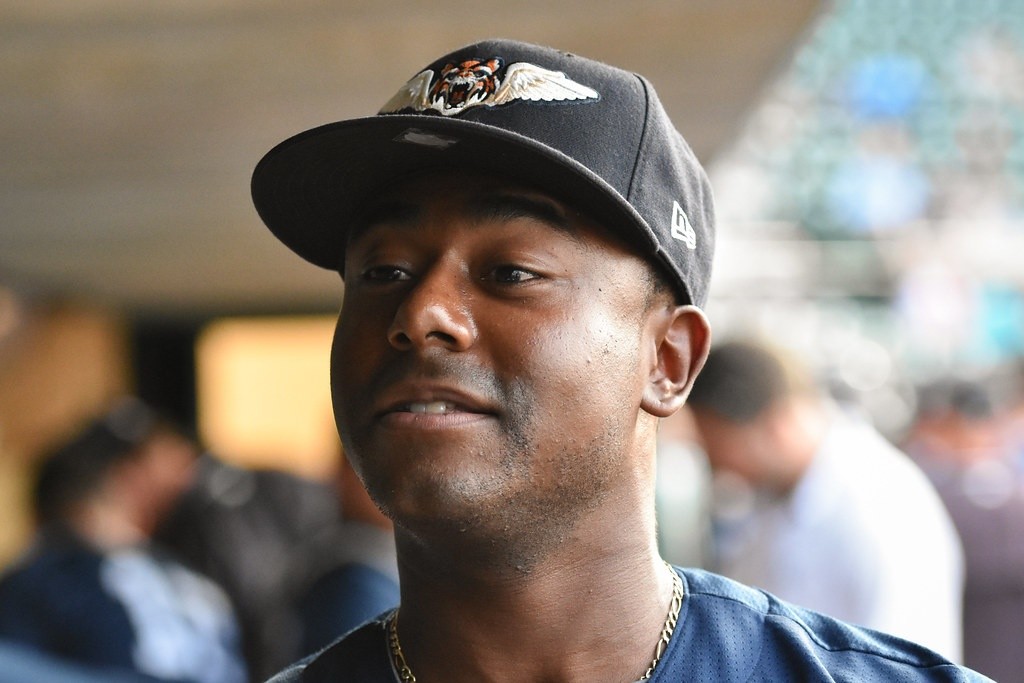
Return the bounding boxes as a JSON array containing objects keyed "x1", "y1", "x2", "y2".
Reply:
[{"x1": 390, "y1": 559, "x2": 683, "y2": 683}]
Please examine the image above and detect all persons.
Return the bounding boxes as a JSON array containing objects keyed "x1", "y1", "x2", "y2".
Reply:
[
  {"x1": 658, "y1": 333, "x2": 1023, "y2": 683},
  {"x1": 0, "y1": 418, "x2": 401, "y2": 683},
  {"x1": 250, "y1": 39, "x2": 1024, "y2": 683}
]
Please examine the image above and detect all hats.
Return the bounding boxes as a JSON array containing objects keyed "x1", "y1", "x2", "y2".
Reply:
[{"x1": 250, "y1": 38, "x2": 719, "y2": 310}]
